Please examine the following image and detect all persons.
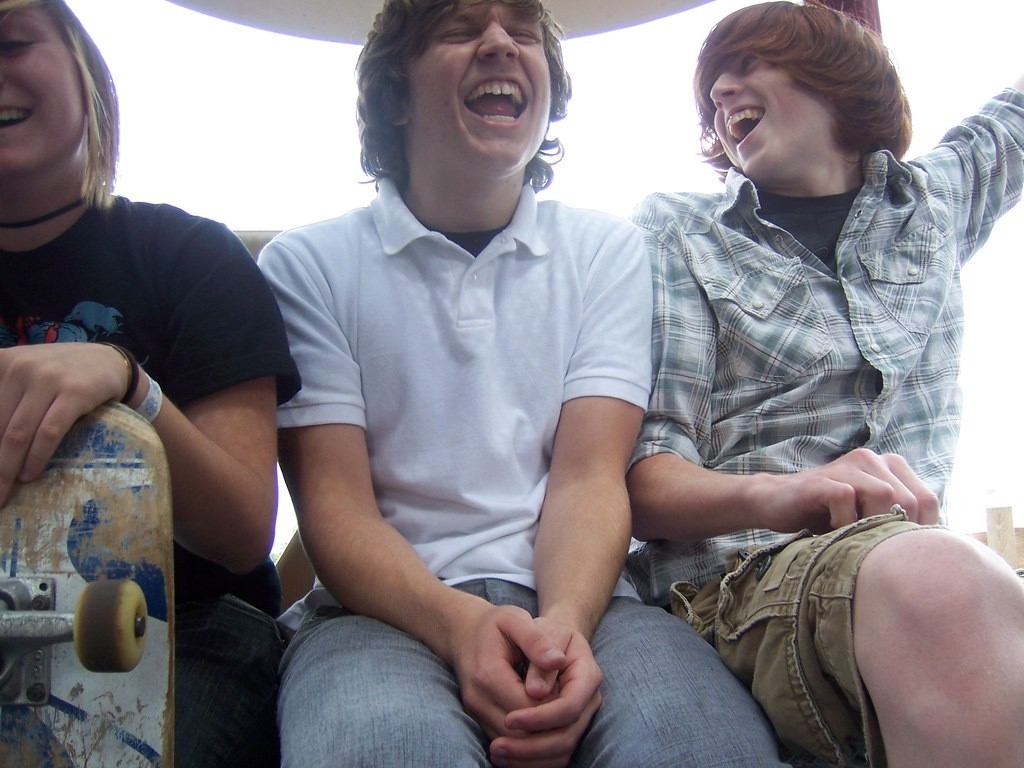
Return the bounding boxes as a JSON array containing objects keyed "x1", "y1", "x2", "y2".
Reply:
[
  {"x1": 255, "y1": 0, "x2": 798, "y2": 768},
  {"x1": 0, "y1": 0, "x2": 302, "y2": 767},
  {"x1": 615, "y1": 2, "x2": 1024, "y2": 767}
]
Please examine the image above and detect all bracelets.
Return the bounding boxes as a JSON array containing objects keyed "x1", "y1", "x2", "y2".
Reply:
[{"x1": 100, "y1": 343, "x2": 162, "y2": 423}]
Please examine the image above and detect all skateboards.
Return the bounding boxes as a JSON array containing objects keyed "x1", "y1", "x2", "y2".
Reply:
[{"x1": 0, "y1": 398, "x2": 175, "y2": 768}]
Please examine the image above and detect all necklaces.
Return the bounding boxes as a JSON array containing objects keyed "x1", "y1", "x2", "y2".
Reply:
[{"x1": 0, "y1": 198, "x2": 87, "y2": 228}]
